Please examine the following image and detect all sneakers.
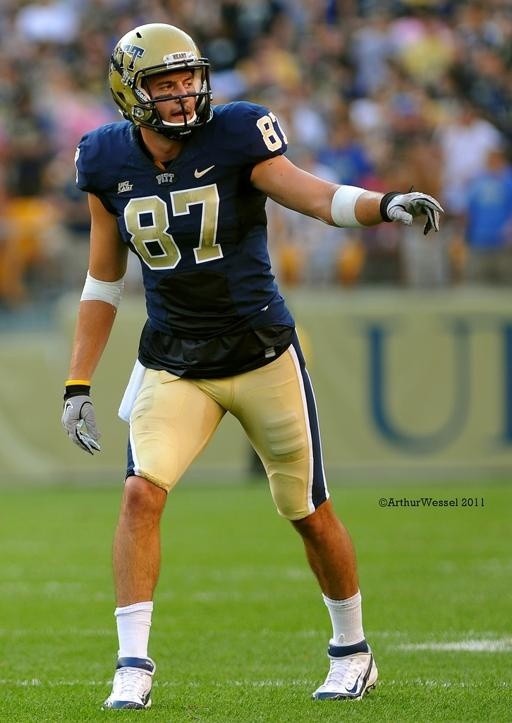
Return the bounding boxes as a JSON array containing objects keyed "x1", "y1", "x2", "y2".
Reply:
[
  {"x1": 104, "y1": 656, "x2": 157, "y2": 710},
  {"x1": 313, "y1": 632, "x2": 378, "y2": 701}
]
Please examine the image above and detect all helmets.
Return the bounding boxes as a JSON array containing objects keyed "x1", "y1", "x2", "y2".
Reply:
[{"x1": 107, "y1": 22, "x2": 215, "y2": 141}]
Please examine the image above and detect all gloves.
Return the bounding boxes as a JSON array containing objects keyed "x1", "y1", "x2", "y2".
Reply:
[
  {"x1": 61, "y1": 380, "x2": 101, "y2": 455},
  {"x1": 380, "y1": 191, "x2": 445, "y2": 235}
]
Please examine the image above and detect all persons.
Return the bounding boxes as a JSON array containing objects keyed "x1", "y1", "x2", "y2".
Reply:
[
  {"x1": 56, "y1": 23, "x2": 445, "y2": 714},
  {"x1": 0, "y1": 0, "x2": 512, "y2": 342}
]
